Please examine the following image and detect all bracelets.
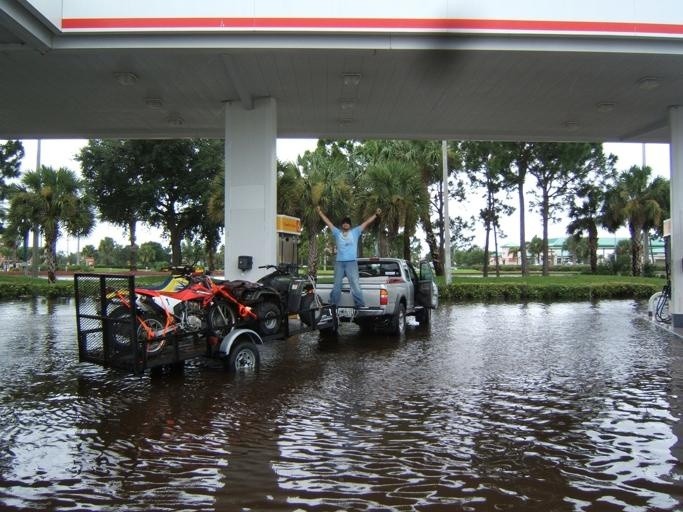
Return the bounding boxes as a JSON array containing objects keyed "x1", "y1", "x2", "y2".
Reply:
[{"x1": 374, "y1": 212, "x2": 379, "y2": 217}]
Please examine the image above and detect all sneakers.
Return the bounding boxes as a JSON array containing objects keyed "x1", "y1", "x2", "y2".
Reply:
[
  {"x1": 354, "y1": 304, "x2": 369, "y2": 310},
  {"x1": 327, "y1": 305, "x2": 338, "y2": 309}
]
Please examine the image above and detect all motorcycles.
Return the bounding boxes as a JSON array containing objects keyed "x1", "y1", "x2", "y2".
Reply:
[{"x1": 95, "y1": 262, "x2": 323, "y2": 358}]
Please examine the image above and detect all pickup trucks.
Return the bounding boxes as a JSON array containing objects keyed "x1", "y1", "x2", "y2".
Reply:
[{"x1": 328, "y1": 257, "x2": 438, "y2": 332}]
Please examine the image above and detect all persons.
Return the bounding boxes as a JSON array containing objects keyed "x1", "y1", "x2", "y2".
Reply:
[{"x1": 314, "y1": 206, "x2": 382, "y2": 309}]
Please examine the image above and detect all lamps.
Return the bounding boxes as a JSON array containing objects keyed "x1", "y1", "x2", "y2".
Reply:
[
  {"x1": 340, "y1": 97, "x2": 355, "y2": 111},
  {"x1": 144, "y1": 99, "x2": 166, "y2": 112},
  {"x1": 597, "y1": 101, "x2": 617, "y2": 114},
  {"x1": 342, "y1": 73, "x2": 362, "y2": 87},
  {"x1": 339, "y1": 119, "x2": 352, "y2": 129},
  {"x1": 563, "y1": 121, "x2": 581, "y2": 133},
  {"x1": 114, "y1": 72, "x2": 137, "y2": 86},
  {"x1": 167, "y1": 114, "x2": 184, "y2": 128},
  {"x1": 636, "y1": 75, "x2": 662, "y2": 91}
]
